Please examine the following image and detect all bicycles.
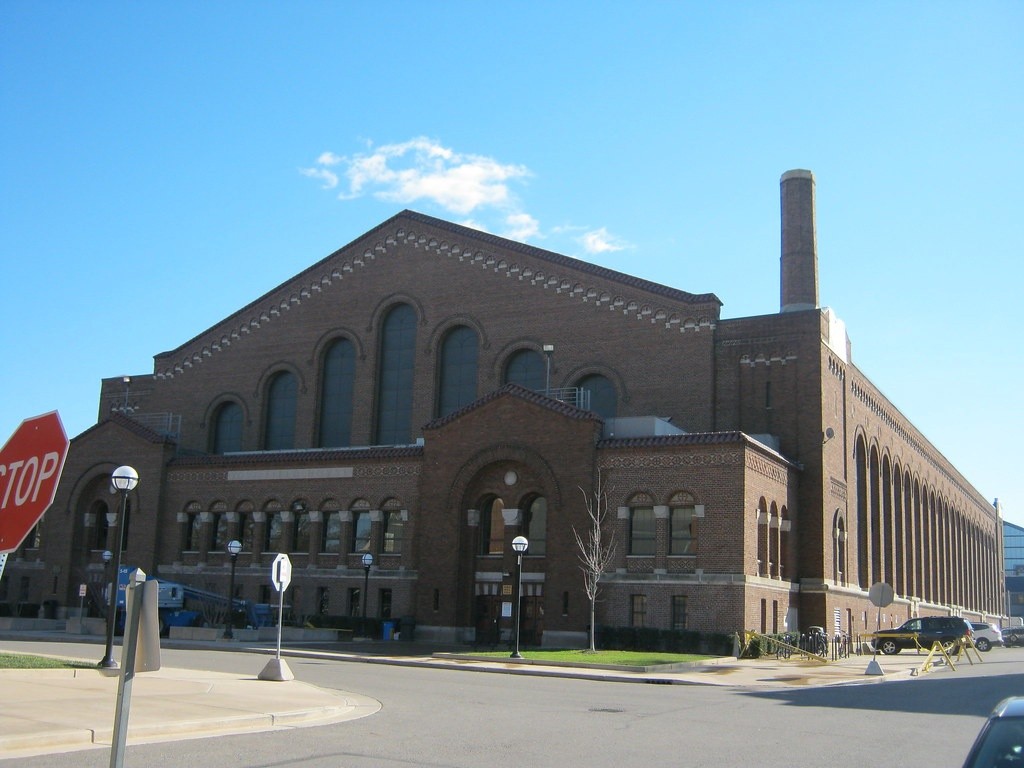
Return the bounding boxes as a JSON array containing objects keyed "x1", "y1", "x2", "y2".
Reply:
[
  {"x1": 837, "y1": 629, "x2": 852, "y2": 658},
  {"x1": 779, "y1": 633, "x2": 796, "y2": 659},
  {"x1": 814, "y1": 630, "x2": 829, "y2": 658}
]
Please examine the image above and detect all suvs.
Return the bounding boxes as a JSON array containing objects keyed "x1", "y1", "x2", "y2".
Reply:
[
  {"x1": 870, "y1": 615, "x2": 974, "y2": 655},
  {"x1": 969, "y1": 621, "x2": 1004, "y2": 652}
]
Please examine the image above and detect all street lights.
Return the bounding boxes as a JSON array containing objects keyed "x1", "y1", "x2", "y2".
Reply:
[
  {"x1": 95, "y1": 465, "x2": 141, "y2": 667},
  {"x1": 510, "y1": 536, "x2": 527, "y2": 659},
  {"x1": 221, "y1": 541, "x2": 243, "y2": 639},
  {"x1": 362, "y1": 553, "x2": 372, "y2": 639}
]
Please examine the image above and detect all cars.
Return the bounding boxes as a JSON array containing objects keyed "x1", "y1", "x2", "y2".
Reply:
[
  {"x1": 959, "y1": 694, "x2": 1024, "y2": 768},
  {"x1": 998, "y1": 626, "x2": 1024, "y2": 648}
]
